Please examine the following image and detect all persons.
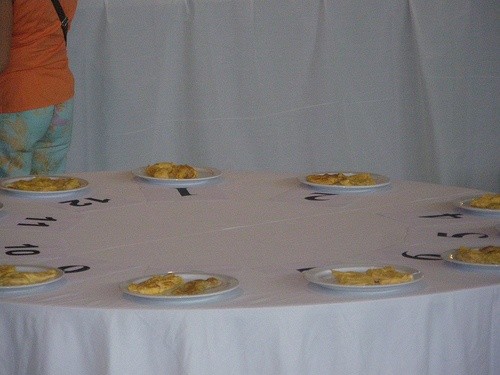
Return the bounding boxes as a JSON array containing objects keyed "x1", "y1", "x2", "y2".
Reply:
[{"x1": 0, "y1": 0, "x2": 78, "y2": 178}]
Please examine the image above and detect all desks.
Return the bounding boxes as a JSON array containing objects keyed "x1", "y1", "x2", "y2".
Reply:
[{"x1": 0, "y1": 171, "x2": 500, "y2": 375}]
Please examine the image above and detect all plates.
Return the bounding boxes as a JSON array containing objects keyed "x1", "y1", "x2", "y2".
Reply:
[
  {"x1": 0, "y1": 264, "x2": 65, "y2": 291},
  {"x1": 456, "y1": 198, "x2": 500, "y2": 216},
  {"x1": 0, "y1": 175, "x2": 91, "y2": 195},
  {"x1": 303, "y1": 262, "x2": 425, "y2": 291},
  {"x1": 440, "y1": 247, "x2": 500, "y2": 270},
  {"x1": 119, "y1": 271, "x2": 239, "y2": 300},
  {"x1": 299, "y1": 171, "x2": 392, "y2": 193},
  {"x1": 132, "y1": 165, "x2": 223, "y2": 184}
]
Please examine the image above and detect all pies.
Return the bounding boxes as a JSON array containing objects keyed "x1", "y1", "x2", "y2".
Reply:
[
  {"x1": 332, "y1": 265, "x2": 410, "y2": 287},
  {"x1": 0, "y1": 262, "x2": 56, "y2": 284},
  {"x1": 5, "y1": 177, "x2": 81, "y2": 192},
  {"x1": 306, "y1": 172, "x2": 375, "y2": 187},
  {"x1": 471, "y1": 192, "x2": 500, "y2": 210},
  {"x1": 127, "y1": 274, "x2": 222, "y2": 295},
  {"x1": 145, "y1": 162, "x2": 197, "y2": 179},
  {"x1": 454, "y1": 244, "x2": 500, "y2": 265}
]
